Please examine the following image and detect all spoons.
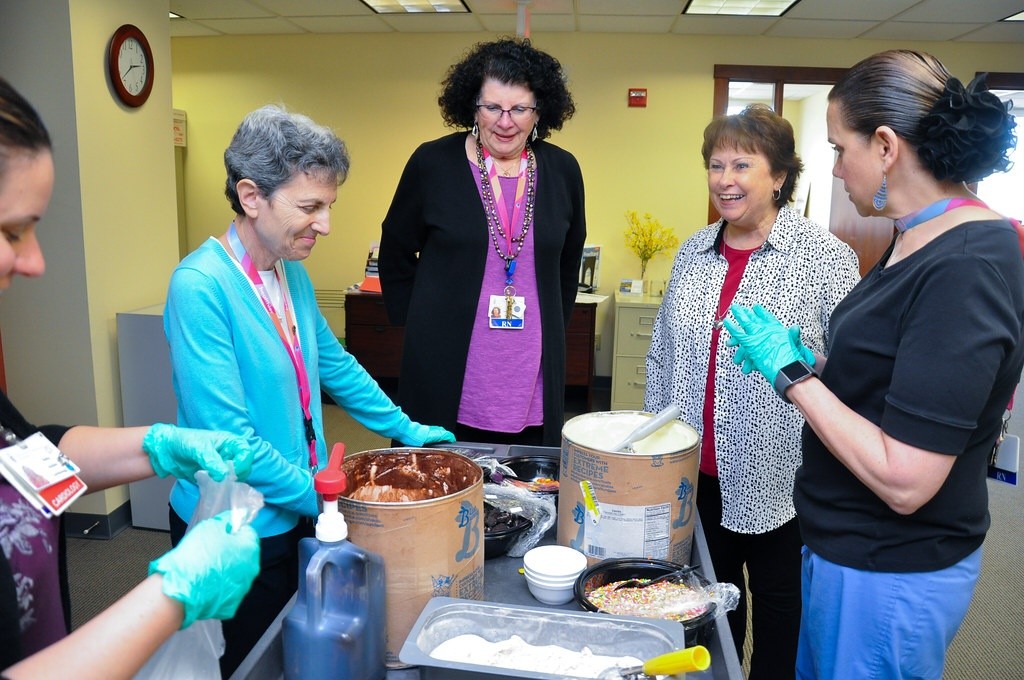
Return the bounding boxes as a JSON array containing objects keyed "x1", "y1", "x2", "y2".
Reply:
[{"x1": 614, "y1": 564, "x2": 702, "y2": 591}]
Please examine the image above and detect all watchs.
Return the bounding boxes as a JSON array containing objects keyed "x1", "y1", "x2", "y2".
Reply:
[{"x1": 774, "y1": 360, "x2": 820, "y2": 404}]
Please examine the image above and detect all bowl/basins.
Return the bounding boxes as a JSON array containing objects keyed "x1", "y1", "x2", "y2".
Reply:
[
  {"x1": 522, "y1": 545, "x2": 587, "y2": 607},
  {"x1": 571, "y1": 558, "x2": 719, "y2": 643},
  {"x1": 481, "y1": 453, "x2": 563, "y2": 497},
  {"x1": 482, "y1": 501, "x2": 532, "y2": 560}
]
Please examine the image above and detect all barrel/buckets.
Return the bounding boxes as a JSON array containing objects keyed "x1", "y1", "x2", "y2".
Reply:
[
  {"x1": 323, "y1": 447, "x2": 485, "y2": 668},
  {"x1": 556, "y1": 410, "x2": 699, "y2": 581}
]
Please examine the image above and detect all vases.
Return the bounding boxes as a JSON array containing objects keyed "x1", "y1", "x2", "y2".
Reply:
[{"x1": 639, "y1": 271, "x2": 651, "y2": 296}]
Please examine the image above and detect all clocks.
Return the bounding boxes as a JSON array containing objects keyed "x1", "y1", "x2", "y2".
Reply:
[{"x1": 110, "y1": 24, "x2": 155, "y2": 105}]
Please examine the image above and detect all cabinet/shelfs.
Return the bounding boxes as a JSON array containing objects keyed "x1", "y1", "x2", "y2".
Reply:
[
  {"x1": 344, "y1": 289, "x2": 599, "y2": 415},
  {"x1": 610, "y1": 290, "x2": 662, "y2": 410}
]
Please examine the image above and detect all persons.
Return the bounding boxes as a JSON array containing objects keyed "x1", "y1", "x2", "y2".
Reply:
[
  {"x1": 0, "y1": 77, "x2": 261, "y2": 680},
  {"x1": 491, "y1": 307, "x2": 501, "y2": 318},
  {"x1": 723, "y1": 51, "x2": 1024, "y2": 680},
  {"x1": 23, "y1": 466, "x2": 50, "y2": 488},
  {"x1": 643, "y1": 110, "x2": 860, "y2": 680},
  {"x1": 378, "y1": 33, "x2": 586, "y2": 448},
  {"x1": 163, "y1": 107, "x2": 456, "y2": 680}
]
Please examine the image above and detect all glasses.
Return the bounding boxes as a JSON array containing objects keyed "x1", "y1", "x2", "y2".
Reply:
[{"x1": 475, "y1": 98, "x2": 537, "y2": 120}]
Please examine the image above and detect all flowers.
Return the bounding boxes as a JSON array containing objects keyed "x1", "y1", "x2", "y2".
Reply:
[
  {"x1": 623, "y1": 209, "x2": 679, "y2": 278},
  {"x1": 914, "y1": 70, "x2": 1020, "y2": 182}
]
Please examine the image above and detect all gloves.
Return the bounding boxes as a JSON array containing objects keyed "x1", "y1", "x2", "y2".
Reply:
[
  {"x1": 425, "y1": 426, "x2": 457, "y2": 445},
  {"x1": 142, "y1": 423, "x2": 252, "y2": 485},
  {"x1": 723, "y1": 303, "x2": 816, "y2": 391},
  {"x1": 148, "y1": 508, "x2": 259, "y2": 631}
]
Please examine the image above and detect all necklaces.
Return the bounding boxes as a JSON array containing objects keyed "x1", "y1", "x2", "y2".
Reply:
[
  {"x1": 714, "y1": 226, "x2": 730, "y2": 331},
  {"x1": 475, "y1": 121, "x2": 535, "y2": 270}
]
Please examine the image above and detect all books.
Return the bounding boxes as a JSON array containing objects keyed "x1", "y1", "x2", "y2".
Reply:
[{"x1": 579, "y1": 245, "x2": 601, "y2": 291}]
